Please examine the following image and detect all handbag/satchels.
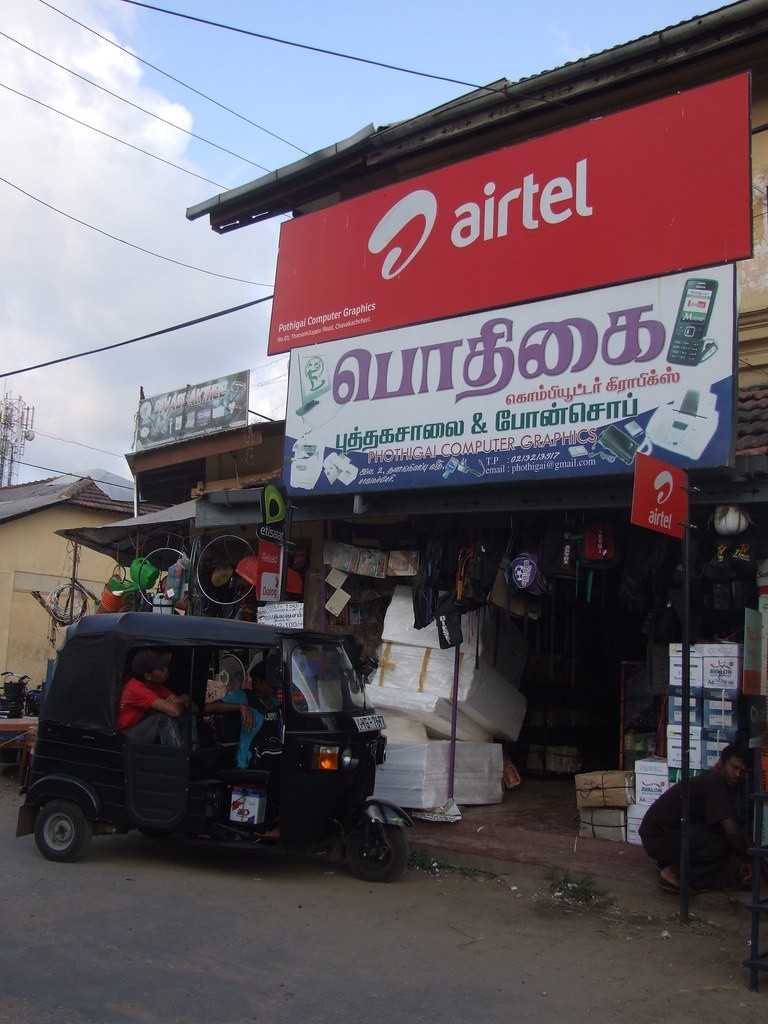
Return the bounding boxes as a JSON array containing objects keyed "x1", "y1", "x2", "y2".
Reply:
[
  {"x1": 504, "y1": 553, "x2": 537, "y2": 591},
  {"x1": 486, "y1": 527, "x2": 542, "y2": 622},
  {"x1": 411, "y1": 537, "x2": 447, "y2": 628},
  {"x1": 435, "y1": 590, "x2": 465, "y2": 649},
  {"x1": 516, "y1": 551, "x2": 548, "y2": 598}
]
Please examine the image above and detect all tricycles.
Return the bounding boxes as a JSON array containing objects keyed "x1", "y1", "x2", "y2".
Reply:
[{"x1": 0, "y1": 672, "x2": 32, "y2": 719}]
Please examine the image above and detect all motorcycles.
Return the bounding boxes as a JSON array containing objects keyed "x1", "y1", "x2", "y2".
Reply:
[{"x1": 15, "y1": 614, "x2": 416, "y2": 883}]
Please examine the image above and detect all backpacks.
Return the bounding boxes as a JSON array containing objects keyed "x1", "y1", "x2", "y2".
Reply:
[
  {"x1": 455, "y1": 543, "x2": 499, "y2": 608},
  {"x1": 577, "y1": 517, "x2": 622, "y2": 570},
  {"x1": 541, "y1": 519, "x2": 584, "y2": 587}
]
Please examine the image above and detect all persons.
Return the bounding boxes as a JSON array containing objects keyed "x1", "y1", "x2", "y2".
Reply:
[
  {"x1": 201, "y1": 659, "x2": 286, "y2": 841},
  {"x1": 637, "y1": 745, "x2": 768, "y2": 895},
  {"x1": 114, "y1": 648, "x2": 201, "y2": 752}
]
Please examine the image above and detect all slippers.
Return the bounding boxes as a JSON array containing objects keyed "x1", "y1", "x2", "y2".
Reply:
[{"x1": 657, "y1": 874, "x2": 707, "y2": 895}]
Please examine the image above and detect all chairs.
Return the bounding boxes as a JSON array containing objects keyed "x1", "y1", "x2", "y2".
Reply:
[
  {"x1": 212, "y1": 710, "x2": 270, "y2": 784},
  {"x1": 158, "y1": 710, "x2": 224, "y2": 795}
]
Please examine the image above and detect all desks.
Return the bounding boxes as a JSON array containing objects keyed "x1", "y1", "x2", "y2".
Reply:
[{"x1": 0, "y1": 719, "x2": 38, "y2": 786}]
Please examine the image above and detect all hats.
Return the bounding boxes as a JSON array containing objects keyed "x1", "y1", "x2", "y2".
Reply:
[{"x1": 131, "y1": 649, "x2": 171, "y2": 675}]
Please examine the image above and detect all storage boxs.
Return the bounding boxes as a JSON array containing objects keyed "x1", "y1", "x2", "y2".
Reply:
[
  {"x1": 230, "y1": 792, "x2": 267, "y2": 824},
  {"x1": 573, "y1": 642, "x2": 743, "y2": 846}
]
